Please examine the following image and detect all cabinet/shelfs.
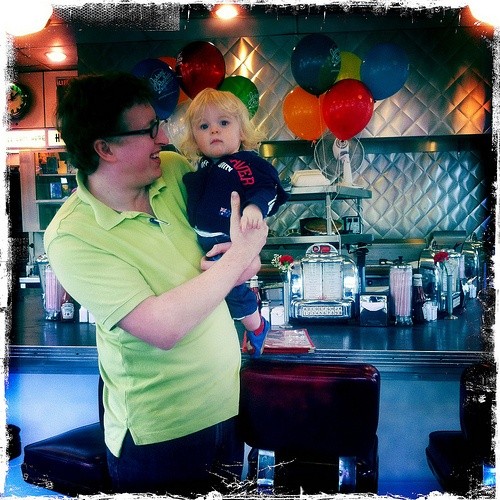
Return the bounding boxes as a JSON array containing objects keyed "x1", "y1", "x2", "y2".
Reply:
[
  {"x1": 33, "y1": 152, "x2": 79, "y2": 232},
  {"x1": 11, "y1": 69, "x2": 78, "y2": 130},
  {"x1": 257, "y1": 186, "x2": 372, "y2": 281}
]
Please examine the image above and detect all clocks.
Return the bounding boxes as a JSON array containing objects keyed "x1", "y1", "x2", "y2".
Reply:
[{"x1": 7, "y1": 83, "x2": 33, "y2": 122}]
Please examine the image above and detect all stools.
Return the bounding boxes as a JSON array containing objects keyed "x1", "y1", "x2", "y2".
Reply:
[
  {"x1": 425, "y1": 360, "x2": 496, "y2": 500},
  {"x1": 238, "y1": 357, "x2": 381, "y2": 500},
  {"x1": 21, "y1": 373, "x2": 114, "y2": 498}
]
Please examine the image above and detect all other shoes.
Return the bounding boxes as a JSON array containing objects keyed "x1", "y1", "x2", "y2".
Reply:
[{"x1": 246, "y1": 316, "x2": 270, "y2": 359}]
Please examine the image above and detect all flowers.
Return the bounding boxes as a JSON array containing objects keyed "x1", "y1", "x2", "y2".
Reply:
[{"x1": 270, "y1": 253, "x2": 292, "y2": 280}]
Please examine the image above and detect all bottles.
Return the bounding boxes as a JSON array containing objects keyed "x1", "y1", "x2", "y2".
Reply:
[
  {"x1": 411, "y1": 274, "x2": 428, "y2": 323},
  {"x1": 260, "y1": 299, "x2": 270, "y2": 324},
  {"x1": 249, "y1": 276, "x2": 261, "y2": 306}
]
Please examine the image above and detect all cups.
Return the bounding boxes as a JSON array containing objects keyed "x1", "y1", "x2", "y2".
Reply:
[
  {"x1": 390, "y1": 265, "x2": 413, "y2": 327},
  {"x1": 35, "y1": 260, "x2": 75, "y2": 323}
]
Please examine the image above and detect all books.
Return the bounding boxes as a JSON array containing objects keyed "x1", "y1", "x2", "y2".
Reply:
[
  {"x1": 48, "y1": 158, "x2": 76, "y2": 198},
  {"x1": 262, "y1": 329, "x2": 315, "y2": 353}
]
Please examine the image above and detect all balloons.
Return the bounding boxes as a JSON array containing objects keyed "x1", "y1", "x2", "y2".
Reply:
[
  {"x1": 459, "y1": 5, "x2": 493, "y2": 41},
  {"x1": 292, "y1": 34, "x2": 340, "y2": 100},
  {"x1": 175, "y1": 41, "x2": 225, "y2": 102},
  {"x1": 282, "y1": 85, "x2": 327, "y2": 144},
  {"x1": 130, "y1": 58, "x2": 180, "y2": 120},
  {"x1": 159, "y1": 56, "x2": 187, "y2": 104},
  {"x1": 361, "y1": 43, "x2": 410, "y2": 100},
  {"x1": 334, "y1": 52, "x2": 362, "y2": 83},
  {"x1": 321, "y1": 79, "x2": 374, "y2": 142},
  {"x1": 218, "y1": 76, "x2": 259, "y2": 119}
]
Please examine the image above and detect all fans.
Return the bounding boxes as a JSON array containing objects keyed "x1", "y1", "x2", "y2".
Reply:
[{"x1": 312, "y1": 131, "x2": 365, "y2": 189}]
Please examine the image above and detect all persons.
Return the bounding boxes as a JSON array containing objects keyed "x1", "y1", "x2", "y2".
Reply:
[
  {"x1": 182, "y1": 87, "x2": 292, "y2": 359},
  {"x1": 43, "y1": 72, "x2": 269, "y2": 496}
]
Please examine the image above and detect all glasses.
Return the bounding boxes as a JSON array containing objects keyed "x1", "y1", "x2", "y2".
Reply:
[{"x1": 105, "y1": 116, "x2": 159, "y2": 139}]
